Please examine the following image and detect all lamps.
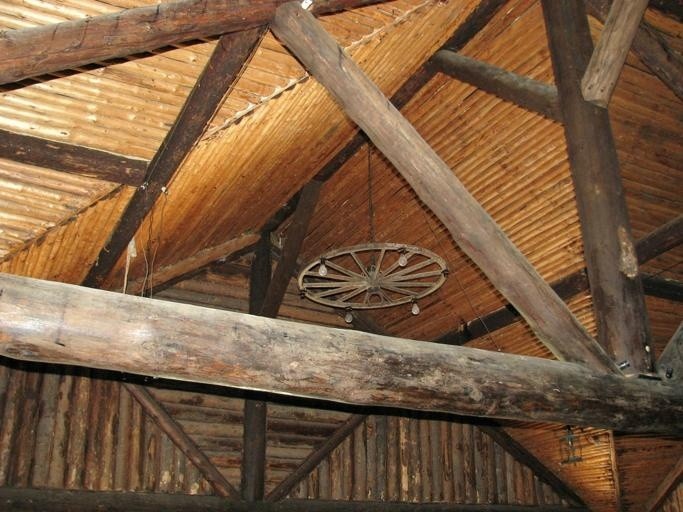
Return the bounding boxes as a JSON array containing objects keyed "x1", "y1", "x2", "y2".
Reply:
[{"x1": 296, "y1": 137, "x2": 449, "y2": 323}]
